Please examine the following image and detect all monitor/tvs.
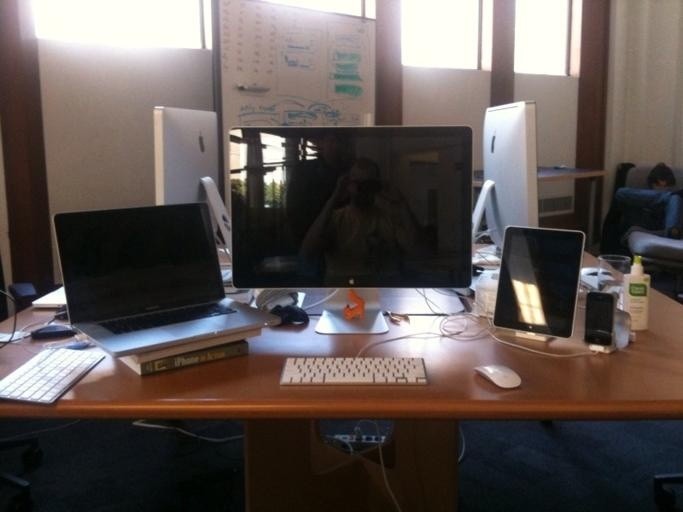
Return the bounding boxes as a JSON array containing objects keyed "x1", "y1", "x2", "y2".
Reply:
[
  {"x1": 151, "y1": 105, "x2": 231, "y2": 284},
  {"x1": 228, "y1": 123, "x2": 474, "y2": 336},
  {"x1": 473, "y1": 98, "x2": 539, "y2": 265}
]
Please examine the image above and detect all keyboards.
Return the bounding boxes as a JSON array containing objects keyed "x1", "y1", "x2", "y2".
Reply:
[
  {"x1": 279, "y1": 355, "x2": 429, "y2": 386},
  {"x1": 0, "y1": 348, "x2": 105, "y2": 405}
]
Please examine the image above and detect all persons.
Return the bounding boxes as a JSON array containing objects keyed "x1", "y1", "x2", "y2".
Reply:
[
  {"x1": 296, "y1": 156, "x2": 424, "y2": 284},
  {"x1": 614, "y1": 162, "x2": 683, "y2": 240}
]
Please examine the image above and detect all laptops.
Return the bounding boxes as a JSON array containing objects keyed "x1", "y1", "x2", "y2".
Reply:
[{"x1": 52, "y1": 201, "x2": 283, "y2": 355}]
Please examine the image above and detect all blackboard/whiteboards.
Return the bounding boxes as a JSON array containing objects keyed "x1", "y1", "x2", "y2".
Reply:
[{"x1": 211, "y1": 1, "x2": 378, "y2": 216}]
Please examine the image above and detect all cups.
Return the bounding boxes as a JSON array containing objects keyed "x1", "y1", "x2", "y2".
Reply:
[{"x1": 597, "y1": 254, "x2": 632, "y2": 312}]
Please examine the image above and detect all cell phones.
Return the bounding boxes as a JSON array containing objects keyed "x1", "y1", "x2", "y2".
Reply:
[{"x1": 584, "y1": 292, "x2": 612, "y2": 347}]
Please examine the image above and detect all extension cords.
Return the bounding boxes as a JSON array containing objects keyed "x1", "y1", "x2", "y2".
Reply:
[{"x1": 334, "y1": 434, "x2": 386, "y2": 444}]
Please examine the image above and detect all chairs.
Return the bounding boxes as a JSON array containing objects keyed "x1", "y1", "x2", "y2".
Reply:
[{"x1": 622, "y1": 165, "x2": 682, "y2": 298}]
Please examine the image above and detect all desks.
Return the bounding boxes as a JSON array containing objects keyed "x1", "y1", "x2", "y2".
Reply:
[
  {"x1": 1, "y1": 244, "x2": 682, "y2": 512},
  {"x1": 472, "y1": 167, "x2": 608, "y2": 251}
]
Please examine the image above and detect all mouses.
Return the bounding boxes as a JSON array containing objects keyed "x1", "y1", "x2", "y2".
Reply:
[
  {"x1": 474, "y1": 364, "x2": 522, "y2": 389},
  {"x1": 31, "y1": 324, "x2": 76, "y2": 339},
  {"x1": 281, "y1": 305, "x2": 309, "y2": 326}
]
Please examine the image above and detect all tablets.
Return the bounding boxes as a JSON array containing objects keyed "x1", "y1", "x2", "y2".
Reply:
[{"x1": 492, "y1": 225, "x2": 586, "y2": 339}]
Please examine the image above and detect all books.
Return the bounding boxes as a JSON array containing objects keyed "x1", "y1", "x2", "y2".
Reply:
[
  {"x1": 119, "y1": 340, "x2": 249, "y2": 377},
  {"x1": 132, "y1": 326, "x2": 262, "y2": 364}
]
